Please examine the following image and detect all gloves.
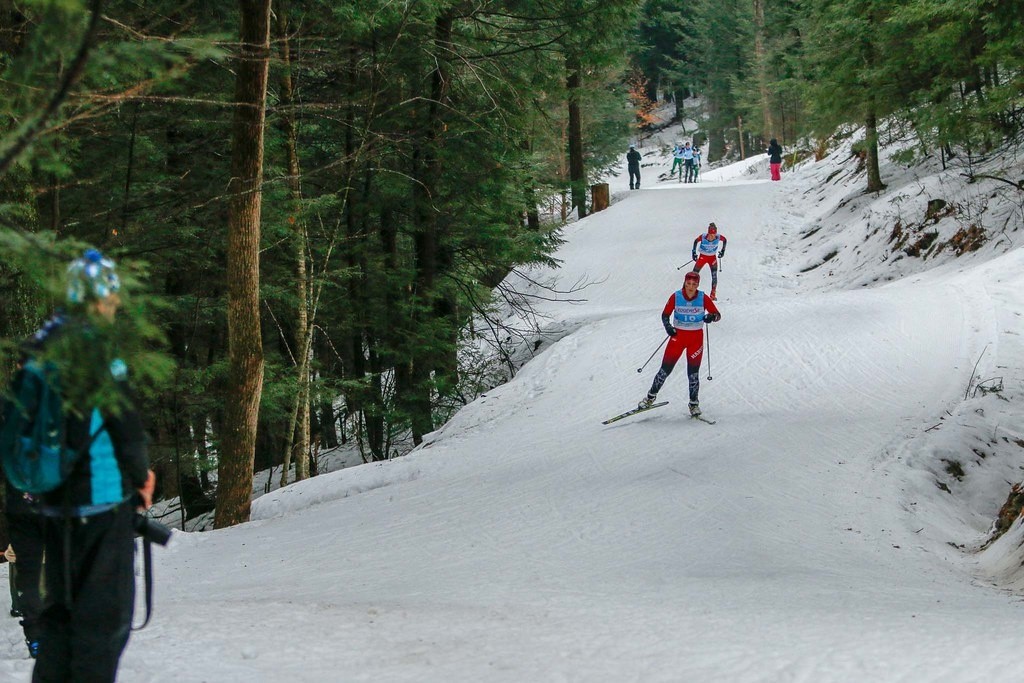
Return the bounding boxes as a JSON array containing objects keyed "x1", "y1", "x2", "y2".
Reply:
[
  {"x1": 703, "y1": 314, "x2": 713, "y2": 323},
  {"x1": 665, "y1": 324, "x2": 676, "y2": 336},
  {"x1": 692, "y1": 252, "x2": 698, "y2": 261},
  {"x1": 718, "y1": 249, "x2": 724, "y2": 258}
]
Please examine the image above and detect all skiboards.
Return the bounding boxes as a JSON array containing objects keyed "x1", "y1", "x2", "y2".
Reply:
[{"x1": 599, "y1": 400, "x2": 717, "y2": 427}]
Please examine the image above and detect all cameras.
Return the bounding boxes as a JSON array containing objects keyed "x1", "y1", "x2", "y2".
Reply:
[{"x1": 132, "y1": 492, "x2": 172, "y2": 547}]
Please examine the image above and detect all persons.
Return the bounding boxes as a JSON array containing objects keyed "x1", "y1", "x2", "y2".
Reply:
[
  {"x1": 670, "y1": 146, "x2": 686, "y2": 182},
  {"x1": 682, "y1": 142, "x2": 696, "y2": 183},
  {"x1": 0, "y1": 248, "x2": 155, "y2": 683},
  {"x1": 692, "y1": 223, "x2": 728, "y2": 300},
  {"x1": 689, "y1": 145, "x2": 701, "y2": 183},
  {"x1": 767, "y1": 138, "x2": 783, "y2": 181},
  {"x1": 638, "y1": 272, "x2": 720, "y2": 415},
  {"x1": 627, "y1": 147, "x2": 641, "y2": 190}
]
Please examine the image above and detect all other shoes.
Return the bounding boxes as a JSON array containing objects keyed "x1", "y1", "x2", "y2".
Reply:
[
  {"x1": 710, "y1": 294, "x2": 717, "y2": 301},
  {"x1": 28, "y1": 640, "x2": 38, "y2": 657},
  {"x1": 12, "y1": 607, "x2": 23, "y2": 616},
  {"x1": 638, "y1": 397, "x2": 656, "y2": 409},
  {"x1": 689, "y1": 403, "x2": 701, "y2": 416}
]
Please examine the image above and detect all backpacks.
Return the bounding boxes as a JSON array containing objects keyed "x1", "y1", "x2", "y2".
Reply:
[{"x1": 0, "y1": 357, "x2": 107, "y2": 496}]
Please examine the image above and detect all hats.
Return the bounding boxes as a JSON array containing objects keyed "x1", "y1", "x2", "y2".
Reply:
[
  {"x1": 65, "y1": 250, "x2": 120, "y2": 305},
  {"x1": 686, "y1": 272, "x2": 700, "y2": 284},
  {"x1": 708, "y1": 222, "x2": 717, "y2": 234}
]
